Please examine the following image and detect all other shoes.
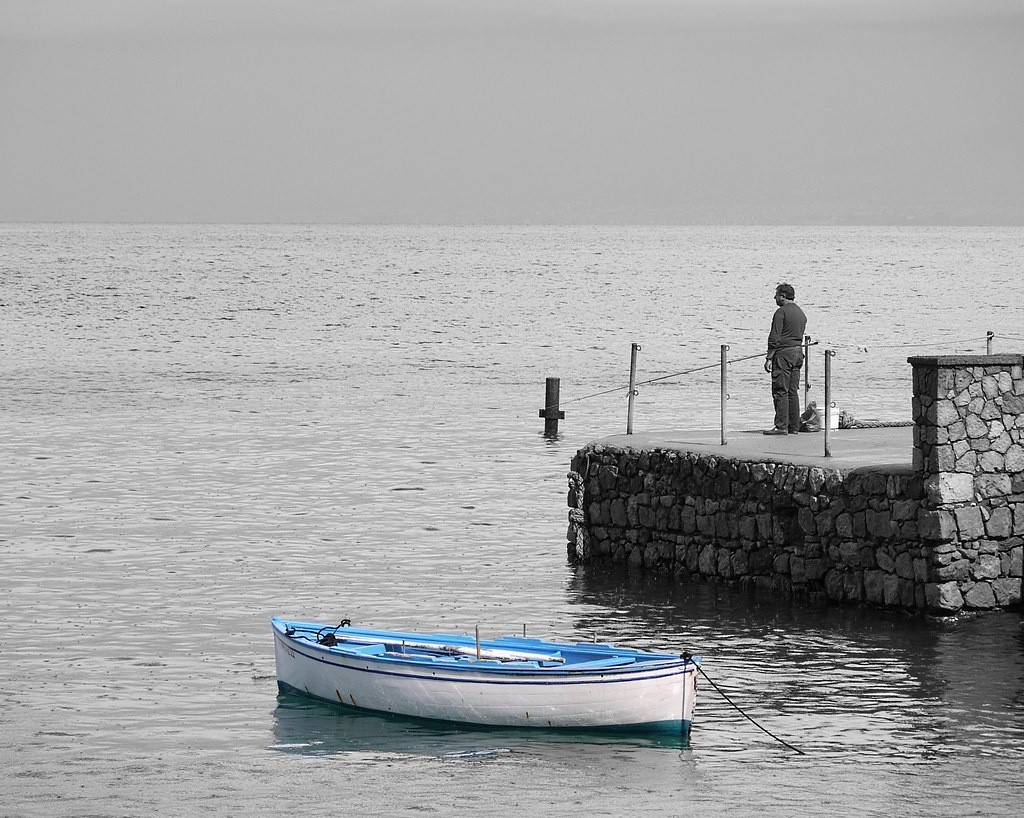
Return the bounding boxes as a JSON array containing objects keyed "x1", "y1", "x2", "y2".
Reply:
[
  {"x1": 789, "y1": 430, "x2": 798, "y2": 434},
  {"x1": 763, "y1": 427, "x2": 788, "y2": 435}
]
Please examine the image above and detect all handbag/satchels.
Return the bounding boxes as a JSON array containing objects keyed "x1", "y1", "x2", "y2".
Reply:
[{"x1": 799, "y1": 401, "x2": 820, "y2": 432}]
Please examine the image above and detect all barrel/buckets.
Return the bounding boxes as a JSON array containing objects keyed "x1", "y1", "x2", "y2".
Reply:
[{"x1": 816, "y1": 408, "x2": 840, "y2": 429}]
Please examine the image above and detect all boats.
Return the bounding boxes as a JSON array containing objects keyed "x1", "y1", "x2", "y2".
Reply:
[{"x1": 270, "y1": 615, "x2": 701, "y2": 736}]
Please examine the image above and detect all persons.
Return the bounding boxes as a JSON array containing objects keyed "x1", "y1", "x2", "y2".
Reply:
[
  {"x1": 762, "y1": 284, "x2": 807, "y2": 435},
  {"x1": 799, "y1": 401, "x2": 821, "y2": 432}
]
